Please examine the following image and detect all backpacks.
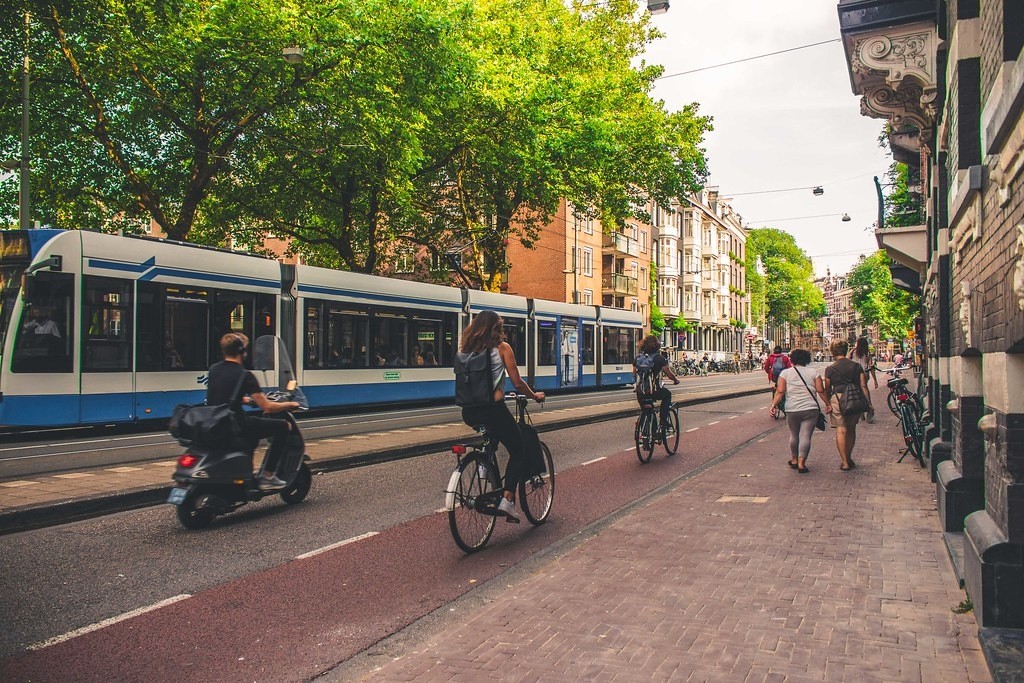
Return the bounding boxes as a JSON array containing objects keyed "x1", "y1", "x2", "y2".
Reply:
[
  {"x1": 634, "y1": 353, "x2": 661, "y2": 394},
  {"x1": 454, "y1": 345, "x2": 503, "y2": 408},
  {"x1": 770, "y1": 356, "x2": 784, "y2": 376},
  {"x1": 829, "y1": 363, "x2": 870, "y2": 416}
]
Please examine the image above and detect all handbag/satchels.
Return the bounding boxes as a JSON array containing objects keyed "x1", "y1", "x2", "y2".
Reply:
[
  {"x1": 171, "y1": 402, "x2": 242, "y2": 446},
  {"x1": 514, "y1": 401, "x2": 547, "y2": 481},
  {"x1": 815, "y1": 413, "x2": 828, "y2": 431}
]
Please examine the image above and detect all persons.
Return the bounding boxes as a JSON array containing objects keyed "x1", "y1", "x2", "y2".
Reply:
[
  {"x1": 769, "y1": 349, "x2": 832, "y2": 472},
  {"x1": 460, "y1": 311, "x2": 546, "y2": 521},
  {"x1": 764, "y1": 346, "x2": 791, "y2": 392},
  {"x1": 561, "y1": 331, "x2": 571, "y2": 384},
  {"x1": 685, "y1": 350, "x2": 752, "y2": 374},
  {"x1": 207, "y1": 333, "x2": 300, "y2": 492},
  {"x1": 824, "y1": 339, "x2": 874, "y2": 469},
  {"x1": 22, "y1": 306, "x2": 62, "y2": 339},
  {"x1": 306, "y1": 345, "x2": 449, "y2": 368},
  {"x1": 848, "y1": 338, "x2": 878, "y2": 419},
  {"x1": 886, "y1": 348, "x2": 913, "y2": 366},
  {"x1": 634, "y1": 336, "x2": 680, "y2": 449}
]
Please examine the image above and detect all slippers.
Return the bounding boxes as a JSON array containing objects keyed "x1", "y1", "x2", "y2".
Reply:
[
  {"x1": 848, "y1": 460, "x2": 854, "y2": 467},
  {"x1": 840, "y1": 466, "x2": 848, "y2": 470}
]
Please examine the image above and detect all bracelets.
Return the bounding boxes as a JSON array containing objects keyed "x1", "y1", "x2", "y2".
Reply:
[{"x1": 773, "y1": 405, "x2": 777, "y2": 410}]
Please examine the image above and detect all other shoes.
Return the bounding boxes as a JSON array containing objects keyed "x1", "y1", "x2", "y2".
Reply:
[
  {"x1": 798, "y1": 466, "x2": 808, "y2": 472},
  {"x1": 567, "y1": 380, "x2": 570, "y2": 383},
  {"x1": 788, "y1": 460, "x2": 798, "y2": 469},
  {"x1": 565, "y1": 381, "x2": 567, "y2": 385},
  {"x1": 659, "y1": 419, "x2": 672, "y2": 427},
  {"x1": 642, "y1": 444, "x2": 651, "y2": 451}
]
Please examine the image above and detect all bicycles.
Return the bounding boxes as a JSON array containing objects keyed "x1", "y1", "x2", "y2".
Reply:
[
  {"x1": 633, "y1": 382, "x2": 680, "y2": 464},
  {"x1": 865, "y1": 361, "x2": 932, "y2": 469},
  {"x1": 664, "y1": 357, "x2": 757, "y2": 376},
  {"x1": 442, "y1": 387, "x2": 554, "y2": 554}
]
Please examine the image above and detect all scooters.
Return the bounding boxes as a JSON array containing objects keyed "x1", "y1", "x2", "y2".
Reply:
[{"x1": 168, "y1": 334, "x2": 313, "y2": 529}]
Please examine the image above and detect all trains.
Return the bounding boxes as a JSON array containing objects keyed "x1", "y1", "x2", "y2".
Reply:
[{"x1": 1, "y1": 227, "x2": 642, "y2": 433}]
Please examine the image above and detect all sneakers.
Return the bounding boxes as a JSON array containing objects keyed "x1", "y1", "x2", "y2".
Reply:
[
  {"x1": 478, "y1": 466, "x2": 488, "y2": 479},
  {"x1": 259, "y1": 475, "x2": 287, "y2": 489},
  {"x1": 498, "y1": 498, "x2": 521, "y2": 520}
]
